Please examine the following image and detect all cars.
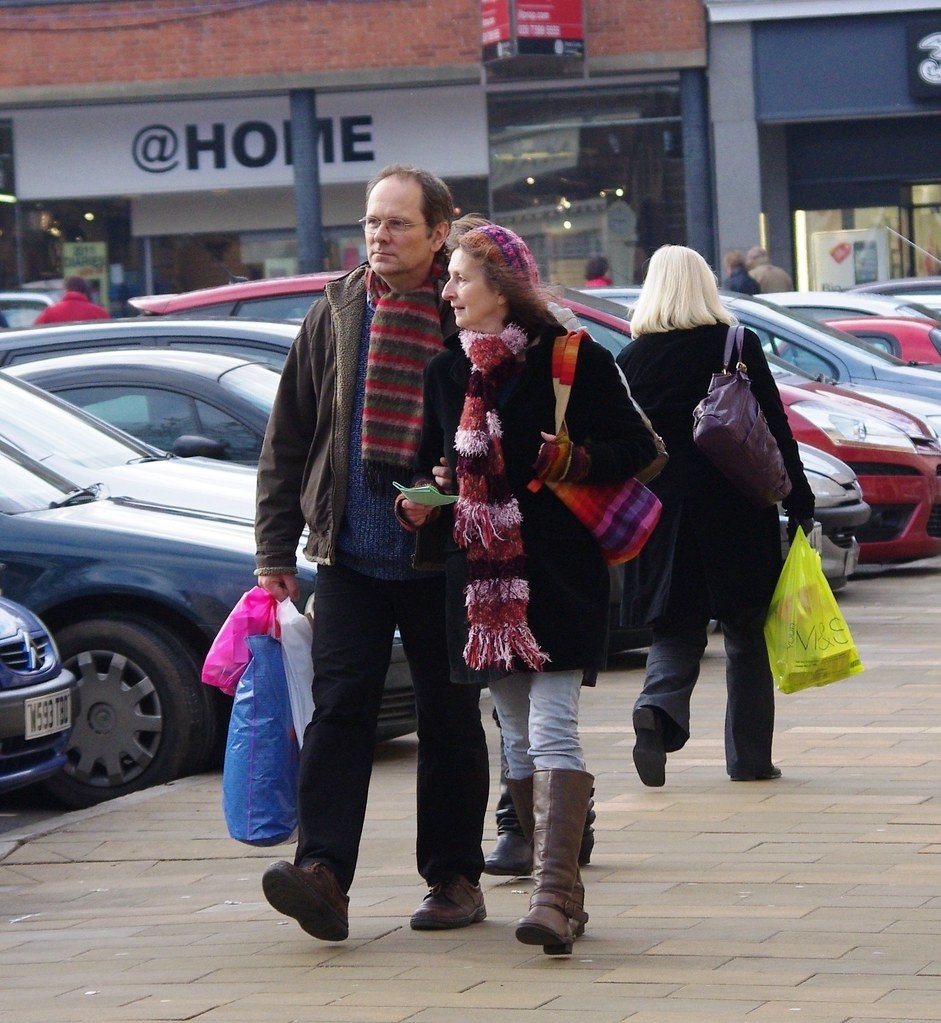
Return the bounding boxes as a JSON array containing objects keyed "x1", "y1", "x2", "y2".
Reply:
[
  {"x1": 0, "y1": 372, "x2": 420, "y2": 811},
  {"x1": 129, "y1": 270, "x2": 941, "y2": 568},
  {"x1": 579, "y1": 277, "x2": 941, "y2": 404},
  {"x1": 0, "y1": 272, "x2": 875, "y2": 653}
]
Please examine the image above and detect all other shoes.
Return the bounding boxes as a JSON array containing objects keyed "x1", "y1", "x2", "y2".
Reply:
[
  {"x1": 633, "y1": 705, "x2": 666, "y2": 786},
  {"x1": 732, "y1": 767, "x2": 782, "y2": 781}
]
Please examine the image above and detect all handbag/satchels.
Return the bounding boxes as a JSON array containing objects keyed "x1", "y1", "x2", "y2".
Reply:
[
  {"x1": 201, "y1": 586, "x2": 279, "y2": 696},
  {"x1": 529, "y1": 329, "x2": 662, "y2": 565},
  {"x1": 275, "y1": 596, "x2": 316, "y2": 750},
  {"x1": 765, "y1": 526, "x2": 863, "y2": 693},
  {"x1": 224, "y1": 634, "x2": 300, "y2": 846},
  {"x1": 693, "y1": 325, "x2": 793, "y2": 509}
]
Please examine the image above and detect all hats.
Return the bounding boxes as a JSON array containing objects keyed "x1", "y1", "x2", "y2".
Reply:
[{"x1": 458, "y1": 225, "x2": 540, "y2": 294}]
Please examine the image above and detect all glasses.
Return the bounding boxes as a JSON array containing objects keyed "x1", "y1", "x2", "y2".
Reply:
[{"x1": 359, "y1": 217, "x2": 431, "y2": 238}]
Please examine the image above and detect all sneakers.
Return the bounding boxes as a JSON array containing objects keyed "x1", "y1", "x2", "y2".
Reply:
[
  {"x1": 263, "y1": 860, "x2": 349, "y2": 941},
  {"x1": 411, "y1": 872, "x2": 486, "y2": 928}
]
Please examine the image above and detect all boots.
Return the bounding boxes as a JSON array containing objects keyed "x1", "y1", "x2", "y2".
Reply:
[
  {"x1": 515, "y1": 768, "x2": 593, "y2": 954},
  {"x1": 579, "y1": 787, "x2": 597, "y2": 865},
  {"x1": 506, "y1": 770, "x2": 589, "y2": 936},
  {"x1": 483, "y1": 708, "x2": 534, "y2": 874}
]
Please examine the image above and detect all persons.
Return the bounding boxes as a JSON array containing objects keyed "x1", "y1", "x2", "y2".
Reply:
[
  {"x1": 746, "y1": 247, "x2": 793, "y2": 292},
  {"x1": 445, "y1": 212, "x2": 596, "y2": 877},
  {"x1": 253, "y1": 165, "x2": 490, "y2": 941},
  {"x1": 393, "y1": 225, "x2": 658, "y2": 955},
  {"x1": 616, "y1": 245, "x2": 815, "y2": 787},
  {"x1": 33, "y1": 277, "x2": 110, "y2": 326},
  {"x1": 722, "y1": 251, "x2": 760, "y2": 295},
  {"x1": 584, "y1": 256, "x2": 612, "y2": 288}
]
